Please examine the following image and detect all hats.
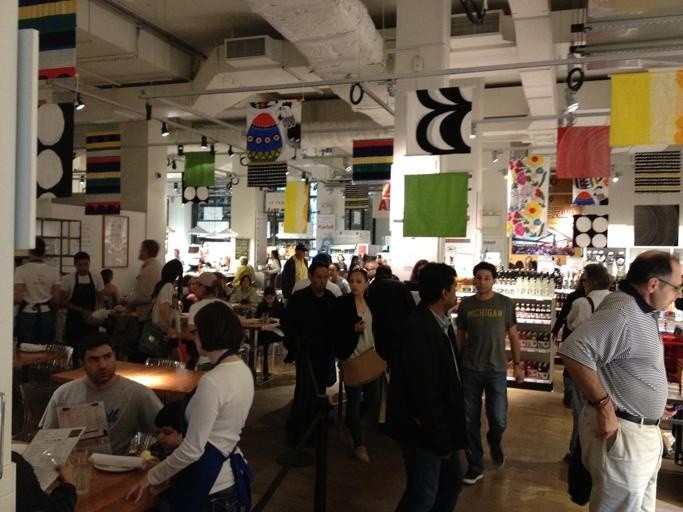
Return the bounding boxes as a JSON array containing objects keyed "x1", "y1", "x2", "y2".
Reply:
[{"x1": 195, "y1": 272, "x2": 218, "y2": 288}]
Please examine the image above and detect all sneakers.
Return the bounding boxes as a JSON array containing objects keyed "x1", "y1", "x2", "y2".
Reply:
[
  {"x1": 486, "y1": 431, "x2": 504, "y2": 466},
  {"x1": 462, "y1": 470, "x2": 485, "y2": 485},
  {"x1": 349, "y1": 444, "x2": 370, "y2": 463}
]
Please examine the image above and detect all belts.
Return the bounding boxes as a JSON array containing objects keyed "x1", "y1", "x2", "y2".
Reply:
[{"x1": 587, "y1": 398, "x2": 660, "y2": 426}]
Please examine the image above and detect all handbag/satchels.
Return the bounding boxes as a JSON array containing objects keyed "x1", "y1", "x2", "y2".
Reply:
[
  {"x1": 338, "y1": 324, "x2": 388, "y2": 389},
  {"x1": 138, "y1": 323, "x2": 179, "y2": 360}
]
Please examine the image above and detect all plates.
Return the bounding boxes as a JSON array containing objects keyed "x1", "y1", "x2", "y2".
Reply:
[{"x1": 94, "y1": 460, "x2": 134, "y2": 473}]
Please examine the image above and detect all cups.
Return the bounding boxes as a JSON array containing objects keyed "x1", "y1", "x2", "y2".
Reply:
[{"x1": 68, "y1": 455, "x2": 94, "y2": 495}]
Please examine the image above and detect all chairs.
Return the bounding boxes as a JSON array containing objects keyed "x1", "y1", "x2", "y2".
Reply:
[{"x1": 7, "y1": 278, "x2": 279, "y2": 512}]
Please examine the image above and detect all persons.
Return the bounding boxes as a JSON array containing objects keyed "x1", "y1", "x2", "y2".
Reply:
[
  {"x1": 125, "y1": 302, "x2": 253, "y2": 512},
  {"x1": 386, "y1": 261, "x2": 468, "y2": 512},
  {"x1": 180, "y1": 251, "x2": 281, "y2": 381},
  {"x1": 13, "y1": 239, "x2": 128, "y2": 358},
  {"x1": 551, "y1": 274, "x2": 583, "y2": 407},
  {"x1": 35, "y1": 335, "x2": 189, "y2": 458},
  {"x1": 11, "y1": 449, "x2": 77, "y2": 512},
  {"x1": 556, "y1": 247, "x2": 682, "y2": 512},
  {"x1": 565, "y1": 260, "x2": 616, "y2": 336},
  {"x1": 121, "y1": 241, "x2": 183, "y2": 363},
  {"x1": 456, "y1": 262, "x2": 525, "y2": 484},
  {"x1": 279, "y1": 244, "x2": 430, "y2": 461}
]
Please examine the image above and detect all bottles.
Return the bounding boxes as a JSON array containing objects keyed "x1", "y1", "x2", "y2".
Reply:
[
  {"x1": 491, "y1": 270, "x2": 555, "y2": 299},
  {"x1": 555, "y1": 272, "x2": 626, "y2": 292},
  {"x1": 504, "y1": 329, "x2": 552, "y2": 349},
  {"x1": 556, "y1": 293, "x2": 569, "y2": 308},
  {"x1": 513, "y1": 302, "x2": 552, "y2": 320},
  {"x1": 454, "y1": 277, "x2": 478, "y2": 294},
  {"x1": 505, "y1": 357, "x2": 549, "y2": 380},
  {"x1": 451, "y1": 297, "x2": 464, "y2": 313}
]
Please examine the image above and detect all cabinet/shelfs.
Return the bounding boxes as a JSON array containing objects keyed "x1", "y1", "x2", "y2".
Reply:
[
  {"x1": 451, "y1": 271, "x2": 476, "y2": 325},
  {"x1": 505, "y1": 263, "x2": 562, "y2": 395},
  {"x1": 557, "y1": 280, "x2": 569, "y2": 306},
  {"x1": 655, "y1": 307, "x2": 681, "y2": 476}
]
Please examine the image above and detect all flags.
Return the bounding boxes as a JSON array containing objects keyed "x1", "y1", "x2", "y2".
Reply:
[
  {"x1": 37, "y1": 67, "x2": 120, "y2": 214},
  {"x1": 402, "y1": 173, "x2": 470, "y2": 237},
  {"x1": 558, "y1": 126, "x2": 614, "y2": 247},
  {"x1": 351, "y1": 138, "x2": 394, "y2": 218},
  {"x1": 181, "y1": 153, "x2": 215, "y2": 204},
  {"x1": 609, "y1": 74, "x2": 682, "y2": 147},
  {"x1": 247, "y1": 101, "x2": 308, "y2": 233},
  {"x1": 407, "y1": 86, "x2": 471, "y2": 156}
]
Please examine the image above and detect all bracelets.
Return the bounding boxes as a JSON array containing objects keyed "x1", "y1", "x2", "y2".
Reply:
[{"x1": 587, "y1": 392, "x2": 610, "y2": 408}]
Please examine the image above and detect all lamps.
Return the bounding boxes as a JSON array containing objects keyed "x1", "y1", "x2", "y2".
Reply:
[
  {"x1": 612, "y1": 172, "x2": 621, "y2": 183},
  {"x1": 490, "y1": 150, "x2": 502, "y2": 163},
  {"x1": 563, "y1": 88, "x2": 579, "y2": 113},
  {"x1": 73, "y1": 91, "x2": 237, "y2": 159}
]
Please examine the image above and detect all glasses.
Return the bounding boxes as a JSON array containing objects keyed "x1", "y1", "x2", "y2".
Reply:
[
  {"x1": 579, "y1": 278, "x2": 590, "y2": 283},
  {"x1": 646, "y1": 275, "x2": 683, "y2": 293},
  {"x1": 189, "y1": 328, "x2": 199, "y2": 336},
  {"x1": 353, "y1": 265, "x2": 368, "y2": 274}
]
